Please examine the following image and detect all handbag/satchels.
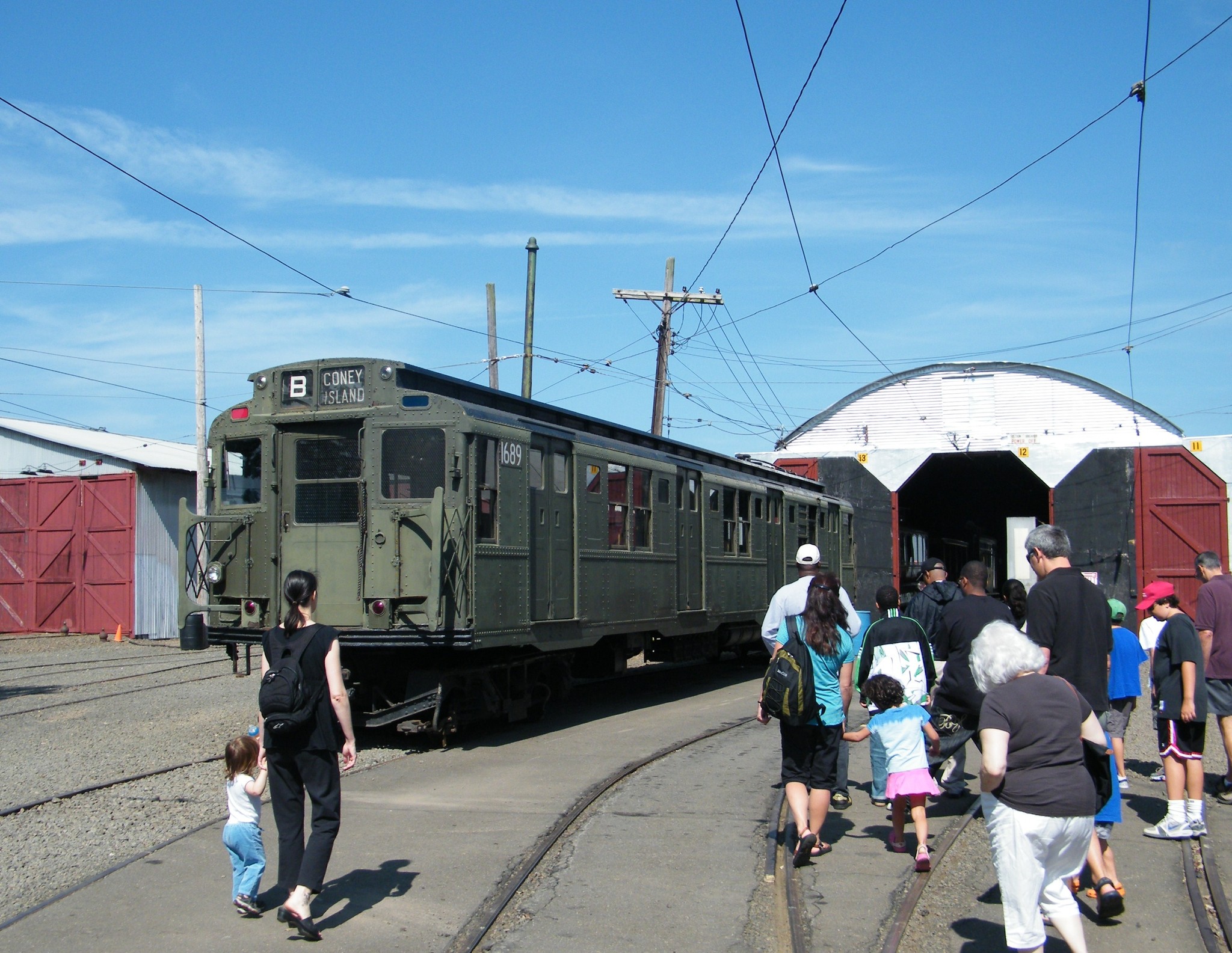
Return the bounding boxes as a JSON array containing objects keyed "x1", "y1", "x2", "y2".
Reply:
[{"x1": 1052, "y1": 675, "x2": 1112, "y2": 813}]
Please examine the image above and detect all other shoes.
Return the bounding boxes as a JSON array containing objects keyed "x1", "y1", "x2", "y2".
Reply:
[
  {"x1": 870, "y1": 797, "x2": 887, "y2": 806},
  {"x1": 949, "y1": 789, "x2": 966, "y2": 798}
]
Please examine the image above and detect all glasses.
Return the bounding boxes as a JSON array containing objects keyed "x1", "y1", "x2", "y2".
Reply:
[
  {"x1": 1147, "y1": 601, "x2": 1162, "y2": 611},
  {"x1": 1026, "y1": 548, "x2": 1040, "y2": 563},
  {"x1": 957, "y1": 576, "x2": 970, "y2": 584}
]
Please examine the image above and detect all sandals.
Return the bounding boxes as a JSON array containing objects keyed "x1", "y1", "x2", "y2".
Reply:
[
  {"x1": 811, "y1": 840, "x2": 834, "y2": 856},
  {"x1": 915, "y1": 844, "x2": 931, "y2": 871},
  {"x1": 792, "y1": 827, "x2": 817, "y2": 868},
  {"x1": 1086, "y1": 883, "x2": 1124, "y2": 897},
  {"x1": 889, "y1": 830, "x2": 907, "y2": 852},
  {"x1": 1065, "y1": 879, "x2": 1081, "y2": 894},
  {"x1": 1094, "y1": 877, "x2": 1125, "y2": 918},
  {"x1": 1040, "y1": 908, "x2": 1053, "y2": 926}
]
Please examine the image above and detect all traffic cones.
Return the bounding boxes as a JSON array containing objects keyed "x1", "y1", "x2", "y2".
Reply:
[{"x1": 113, "y1": 624, "x2": 125, "y2": 642}]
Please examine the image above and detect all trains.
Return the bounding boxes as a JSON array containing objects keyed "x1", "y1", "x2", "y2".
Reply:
[{"x1": 178, "y1": 357, "x2": 858, "y2": 745}]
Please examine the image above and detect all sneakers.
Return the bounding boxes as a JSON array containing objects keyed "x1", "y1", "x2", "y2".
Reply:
[
  {"x1": 1216, "y1": 775, "x2": 1232, "y2": 793},
  {"x1": 1217, "y1": 787, "x2": 1232, "y2": 804},
  {"x1": 1144, "y1": 815, "x2": 1193, "y2": 838},
  {"x1": 833, "y1": 794, "x2": 852, "y2": 809},
  {"x1": 1116, "y1": 774, "x2": 1128, "y2": 788},
  {"x1": 234, "y1": 893, "x2": 265, "y2": 918},
  {"x1": 1150, "y1": 766, "x2": 1165, "y2": 780},
  {"x1": 1189, "y1": 818, "x2": 1207, "y2": 837}
]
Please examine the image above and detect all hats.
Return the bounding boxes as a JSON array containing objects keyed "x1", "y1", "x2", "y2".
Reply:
[
  {"x1": 1135, "y1": 581, "x2": 1173, "y2": 609},
  {"x1": 796, "y1": 543, "x2": 821, "y2": 565},
  {"x1": 916, "y1": 557, "x2": 949, "y2": 581},
  {"x1": 1108, "y1": 598, "x2": 1127, "y2": 620}
]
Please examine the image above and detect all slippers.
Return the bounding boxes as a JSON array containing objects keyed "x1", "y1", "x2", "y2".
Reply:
[{"x1": 278, "y1": 907, "x2": 321, "y2": 940}]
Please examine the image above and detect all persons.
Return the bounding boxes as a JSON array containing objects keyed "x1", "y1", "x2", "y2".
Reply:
[
  {"x1": 855, "y1": 585, "x2": 937, "y2": 807},
  {"x1": 1068, "y1": 733, "x2": 1125, "y2": 897},
  {"x1": 1139, "y1": 615, "x2": 1167, "y2": 780},
  {"x1": 842, "y1": 674, "x2": 941, "y2": 871},
  {"x1": 222, "y1": 735, "x2": 269, "y2": 913},
  {"x1": 1193, "y1": 551, "x2": 1232, "y2": 804},
  {"x1": 969, "y1": 619, "x2": 1107, "y2": 953},
  {"x1": 757, "y1": 573, "x2": 855, "y2": 868},
  {"x1": 1025, "y1": 525, "x2": 1125, "y2": 920},
  {"x1": 905, "y1": 557, "x2": 1028, "y2": 813},
  {"x1": 761, "y1": 544, "x2": 861, "y2": 810},
  {"x1": 1106, "y1": 599, "x2": 1148, "y2": 789},
  {"x1": 1135, "y1": 582, "x2": 1208, "y2": 839},
  {"x1": 257, "y1": 570, "x2": 356, "y2": 942}
]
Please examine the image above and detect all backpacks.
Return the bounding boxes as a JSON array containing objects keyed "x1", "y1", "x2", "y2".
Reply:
[
  {"x1": 258, "y1": 625, "x2": 327, "y2": 739},
  {"x1": 759, "y1": 615, "x2": 820, "y2": 726}
]
